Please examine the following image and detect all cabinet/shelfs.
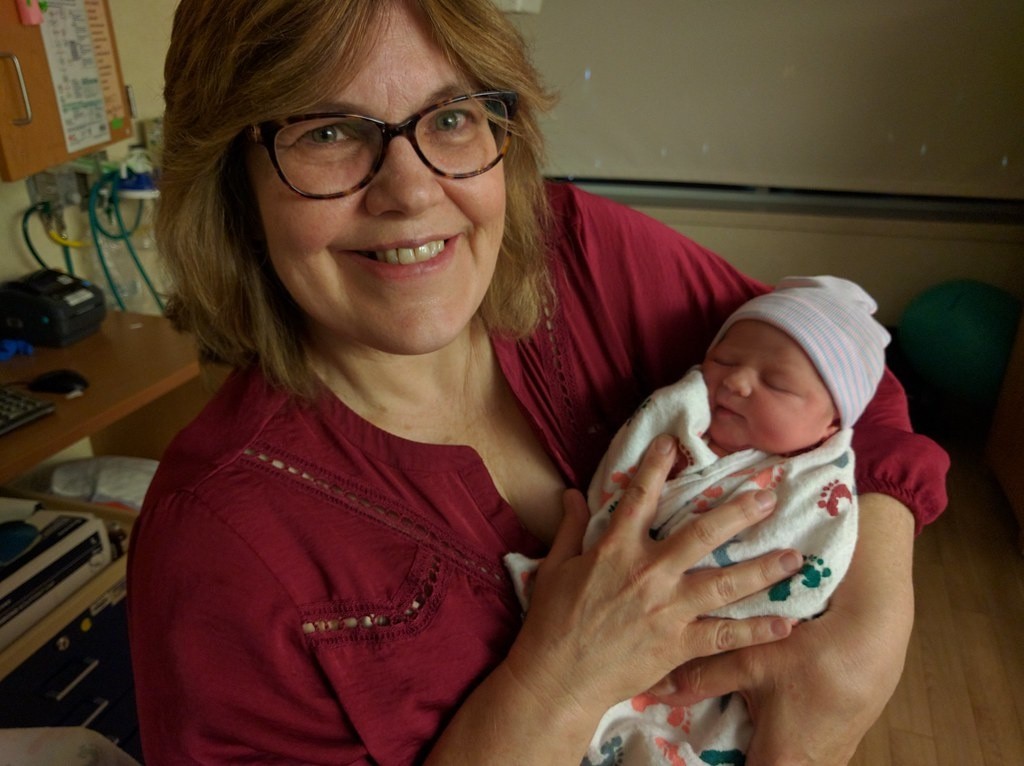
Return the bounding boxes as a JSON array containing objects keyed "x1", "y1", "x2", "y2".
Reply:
[
  {"x1": 0, "y1": 0, "x2": 135, "y2": 184},
  {"x1": 0, "y1": 491, "x2": 146, "y2": 766}
]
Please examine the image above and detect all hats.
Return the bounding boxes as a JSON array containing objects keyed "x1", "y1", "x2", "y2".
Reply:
[{"x1": 708, "y1": 273, "x2": 892, "y2": 433}]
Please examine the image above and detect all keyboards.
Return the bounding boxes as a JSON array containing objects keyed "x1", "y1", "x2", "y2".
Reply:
[{"x1": 0, "y1": 388, "x2": 55, "y2": 438}]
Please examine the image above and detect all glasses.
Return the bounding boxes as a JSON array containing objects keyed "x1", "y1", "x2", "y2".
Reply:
[{"x1": 235, "y1": 89, "x2": 520, "y2": 201}]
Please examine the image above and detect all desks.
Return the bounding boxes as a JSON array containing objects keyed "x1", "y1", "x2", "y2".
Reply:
[{"x1": 0, "y1": 306, "x2": 234, "y2": 489}]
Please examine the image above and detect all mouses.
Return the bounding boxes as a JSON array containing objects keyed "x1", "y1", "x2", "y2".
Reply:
[{"x1": 29, "y1": 369, "x2": 89, "y2": 395}]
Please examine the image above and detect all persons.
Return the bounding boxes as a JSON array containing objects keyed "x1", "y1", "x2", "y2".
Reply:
[
  {"x1": 558, "y1": 275, "x2": 890, "y2": 766},
  {"x1": 124, "y1": 1, "x2": 951, "y2": 766}
]
